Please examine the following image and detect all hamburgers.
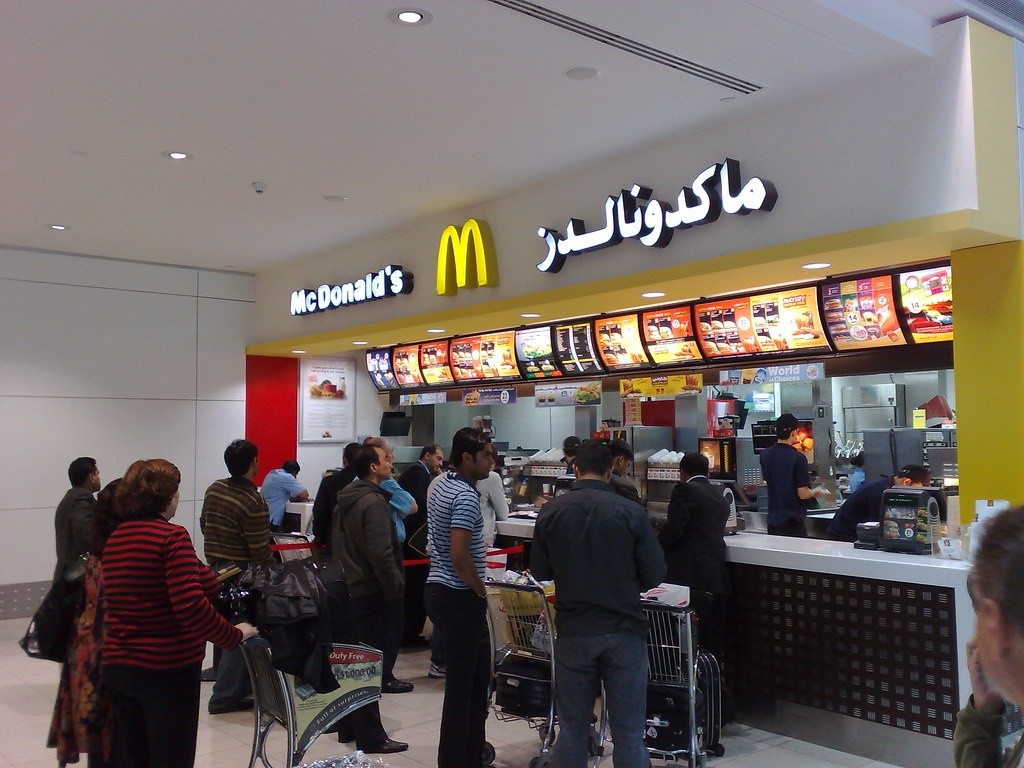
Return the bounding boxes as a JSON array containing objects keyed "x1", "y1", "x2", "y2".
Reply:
[
  {"x1": 599, "y1": 332, "x2": 623, "y2": 365},
  {"x1": 700, "y1": 320, "x2": 743, "y2": 353},
  {"x1": 452, "y1": 350, "x2": 472, "y2": 362},
  {"x1": 465, "y1": 393, "x2": 479, "y2": 405},
  {"x1": 823, "y1": 298, "x2": 847, "y2": 333},
  {"x1": 883, "y1": 521, "x2": 899, "y2": 538},
  {"x1": 648, "y1": 325, "x2": 672, "y2": 339}
]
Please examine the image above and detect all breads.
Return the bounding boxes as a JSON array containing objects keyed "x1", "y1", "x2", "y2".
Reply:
[{"x1": 793, "y1": 438, "x2": 813, "y2": 452}]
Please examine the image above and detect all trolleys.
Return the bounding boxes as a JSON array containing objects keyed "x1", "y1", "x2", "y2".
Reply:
[
  {"x1": 483, "y1": 577, "x2": 725, "y2": 768},
  {"x1": 238, "y1": 637, "x2": 384, "y2": 768},
  {"x1": 269, "y1": 531, "x2": 318, "y2": 568}
]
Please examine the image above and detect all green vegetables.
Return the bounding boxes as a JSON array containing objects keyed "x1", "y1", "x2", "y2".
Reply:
[{"x1": 576, "y1": 382, "x2": 600, "y2": 402}]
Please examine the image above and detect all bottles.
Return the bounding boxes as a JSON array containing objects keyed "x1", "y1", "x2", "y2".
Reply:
[
  {"x1": 518, "y1": 478, "x2": 529, "y2": 496},
  {"x1": 913, "y1": 407, "x2": 926, "y2": 429},
  {"x1": 338, "y1": 377, "x2": 346, "y2": 395},
  {"x1": 728, "y1": 366, "x2": 766, "y2": 385}
]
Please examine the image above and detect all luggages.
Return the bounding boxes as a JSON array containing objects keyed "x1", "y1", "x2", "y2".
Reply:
[{"x1": 646, "y1": 645, "x2": 728, "y2": 759}]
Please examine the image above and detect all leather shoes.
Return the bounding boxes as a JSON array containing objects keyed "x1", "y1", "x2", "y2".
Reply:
[
  {"x1": 362, "y1": 739, "x2": 409, "y2": 754},
  {"x1": 380, "y1": 680, "x2": 415, "y2": 693}
]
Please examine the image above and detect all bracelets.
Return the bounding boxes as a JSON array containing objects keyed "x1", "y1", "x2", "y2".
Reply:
[{"x1": 477, "y1": 592, "x2": 487, "y2": 599}]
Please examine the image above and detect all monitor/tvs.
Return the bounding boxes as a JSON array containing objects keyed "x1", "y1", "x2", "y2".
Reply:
[
  {"x1": 893, "y1": 485, "x2": 948, "y2": 524},
  {"x1": 709, "y1": 478, "x2": 754, "y2": 507},
  {"x1": 380, "y1": 416, "x2": 412, "y2": 437}
]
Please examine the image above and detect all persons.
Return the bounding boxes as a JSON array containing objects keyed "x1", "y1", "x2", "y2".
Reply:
[
  {"x1": 53, "y1": 457, "x2": 101, "y2": 587},
  {"x1": 312, "y1": 437, "x2": 418, "y2": 754},
  {"x1": 423, "y1": 428, "x2": 494, "y2": 768},
  {"x1": 953, "y1": 505, "x2": 1024, "y2": 768},
  {"x1": 397, "y1": 444, "x2": 443, "y2": 649},
  {"x1": 476, "y1": 444, "x2": 509, "y2": 548},
  {"x1": 607, "y1": 439, "x2": 643, "y2": 505},
  {"x1": 50, "y1": 462, "x2": 258, "y2": 768},
  {"x1": 826, "y1": 463, "x2": 930, "y2": 543},
  {"x1": 849, "y1": 451, "x2": 865, "y2": 494},
  {"x1": 200, "y1": 439, "x2": 276, "y2": 715},
  {"x1": 760, "y1": 413, "x2": 822, "y2": 537},
  {"x1": 261, "y1": 460, "x2": 309, "y2": 534},
  {"x1": 529, "y1": 439, "x2": 667, "y2": 768},
  {"x1": 657, "y1": 452, "x2": 731, "y2": 756},
  {"x1": 562, "y1": 436, "x2": 581, "y2": 474}
]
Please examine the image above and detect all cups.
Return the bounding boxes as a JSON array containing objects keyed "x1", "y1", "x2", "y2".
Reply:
[
  {"x1": 543, "y1": 484, "x2": 550, "y2": 496},
  {"x1": 530, "y1": 447, "x2": 565, "y2": 461},
  {"x1": 648, "y1": 449, "x2": 685, "y2": 463},
  {"x1": 946, "y1": 495, "x2": 960, "y2": 540}
]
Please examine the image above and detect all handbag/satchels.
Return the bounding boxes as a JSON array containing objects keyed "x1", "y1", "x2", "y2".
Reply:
[
  {"x1": 494, "y1": 653, "x2": 558, "y2": 718},
  {"x1": 21, "y1": 546, "x2": 90, "y2": 664}
]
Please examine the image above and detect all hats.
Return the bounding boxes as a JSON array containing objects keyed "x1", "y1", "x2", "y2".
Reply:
[
  {"x1": 901, "y1": 464, "x2": 930, "y2": 486},
  {"x1": 776, "y1": 412, "x2": 799, "y2": 430},
  {"x1": 851, "y1": 451, "x2": 865, "y2": 465},
  {"x1": 563, "y1": 437, "x2": 581, "y2": 451}
]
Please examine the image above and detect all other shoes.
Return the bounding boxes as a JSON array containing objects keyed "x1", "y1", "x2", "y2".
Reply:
[
  {"x1": 480, "y1": 739, "x2": 496, "y2": 766},
  {"x1": 427, "y1": 660, "x2": 446, "y2": 678},
  {"x1": 402, "y1": 634, "x2": 429, "y2": 650},
  {"x1": 209, "y1": 697, "x2": 254, "y2": 714}
]
